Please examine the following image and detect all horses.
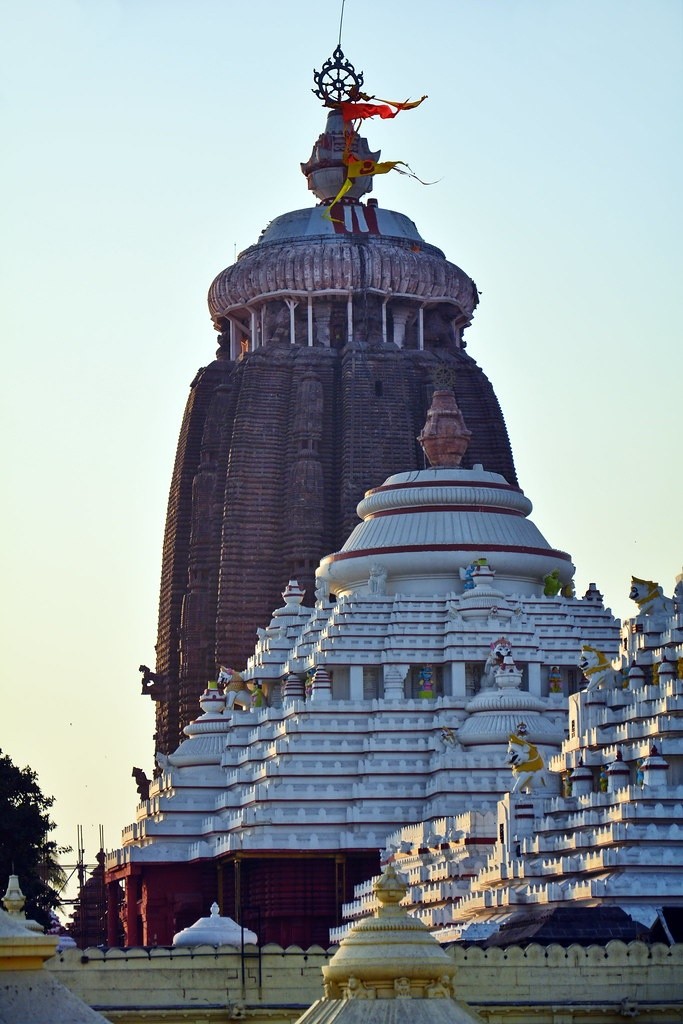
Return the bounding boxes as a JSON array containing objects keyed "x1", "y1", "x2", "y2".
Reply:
[{"x1": 217, "y1": 666, "x2": 254, "y2": 712}]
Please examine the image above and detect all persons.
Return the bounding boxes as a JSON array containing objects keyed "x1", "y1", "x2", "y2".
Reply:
[
  {"x1": 599, "y1": 765, "x2": 608, "y2": 791},
  {"x1": 305, "y1": 672, "x2": 313, "y2": 697},
  {"x1": 635, "y1": 758, "x2": 644, "y2": 786},
  {"x1": 549, "y1": 667, "x2": 564, "y2": 692},
  {"x1": 565, "y1": 768, "x2": 574, "y2": 797},
  {"x1": 250, "y1": 683, "x2": 268, "y2": 707}
]
